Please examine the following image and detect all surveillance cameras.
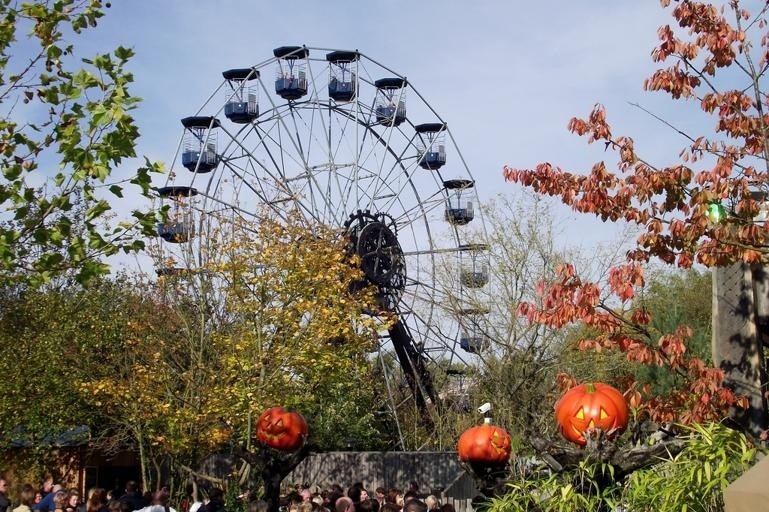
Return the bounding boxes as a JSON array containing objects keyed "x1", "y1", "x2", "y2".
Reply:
[{"x1": 478, "y1": 402, "x2": 491, "y2": 414}]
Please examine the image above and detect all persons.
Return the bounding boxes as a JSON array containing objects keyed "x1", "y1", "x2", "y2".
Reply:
[{"x1": 0, "y1": 472, "x2": 460, "y2": 511}]
